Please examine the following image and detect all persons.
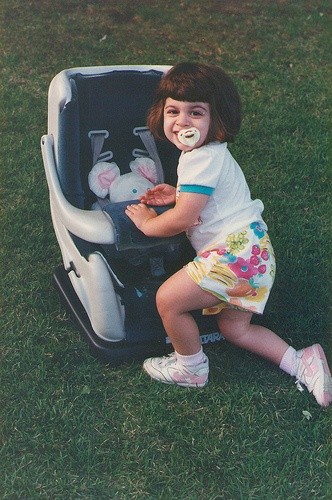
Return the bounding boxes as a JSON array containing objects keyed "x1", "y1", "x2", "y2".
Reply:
[{"x1": 124, "y1": 61, "x2": 332, "y2": 409}]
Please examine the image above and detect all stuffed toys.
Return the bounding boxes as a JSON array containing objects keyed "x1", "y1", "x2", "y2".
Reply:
[{"x1": 88, "y1": 159, "x2": 174, "y2": 267}]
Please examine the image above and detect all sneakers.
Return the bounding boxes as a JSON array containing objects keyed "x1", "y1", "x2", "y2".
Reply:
[
  {"x1": 142, "y1": 352, "x2": 210, "y2": 387},
  {"x1": 295, "y1": 343, "x2": 332, "y2": 407}
]
